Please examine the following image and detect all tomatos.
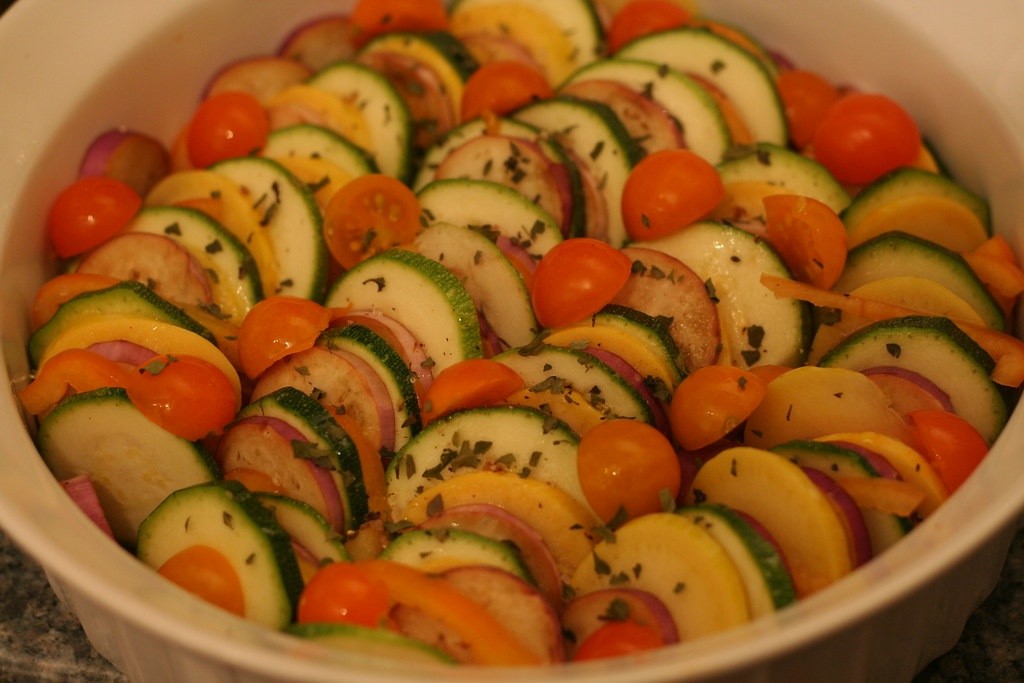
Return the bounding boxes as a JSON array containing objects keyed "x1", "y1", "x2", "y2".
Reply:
[{"x1": 51, "y1": 0, "x2": 992, "y2": 667}]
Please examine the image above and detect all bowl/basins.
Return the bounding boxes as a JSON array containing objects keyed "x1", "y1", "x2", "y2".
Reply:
[{"x1": 0, "y1": 0, "x2": 1024, "y2": 681}]
